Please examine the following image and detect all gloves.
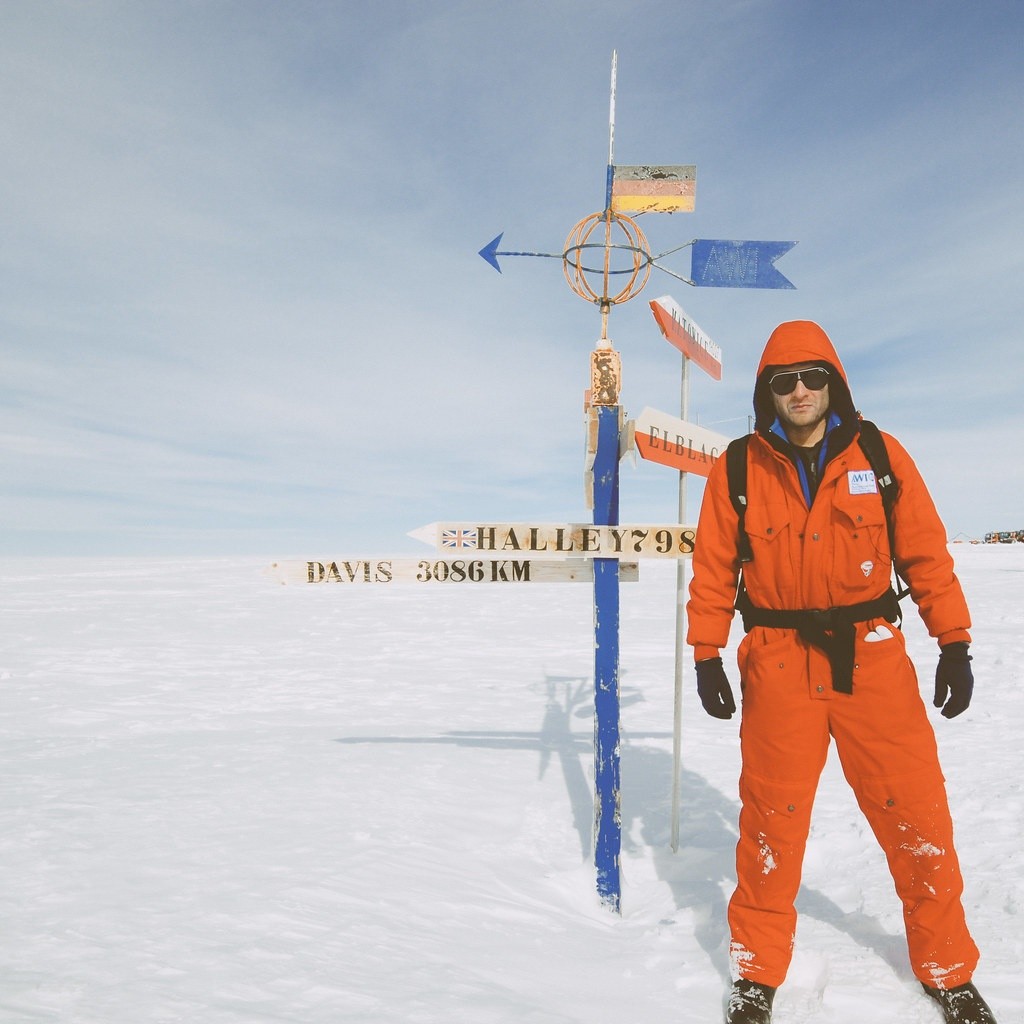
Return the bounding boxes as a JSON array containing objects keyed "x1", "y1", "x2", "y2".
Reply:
[
  {"x1": 933, "y1": 642, "x2": 975, "y2": 719},
  {"x1": 693, "y1": 656, "x2": 737, "y2": 720}
]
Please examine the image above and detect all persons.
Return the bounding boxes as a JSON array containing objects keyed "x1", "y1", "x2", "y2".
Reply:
[{"x1": 686, "y1": 320, "x2": 998, "y2": 1024}]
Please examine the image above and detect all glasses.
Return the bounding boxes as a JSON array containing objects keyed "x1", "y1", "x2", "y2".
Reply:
[{"x1": 766, "y1": 366, "x2": 833, "y2": 395}]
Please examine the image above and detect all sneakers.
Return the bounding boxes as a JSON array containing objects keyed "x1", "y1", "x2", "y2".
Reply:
[
  {"x1": 921, "y1": 982, "x2": 997, "y2": 1024},
  {"x1": 728, "y1": 979, "x2": 777, "y2": 1024}
]
